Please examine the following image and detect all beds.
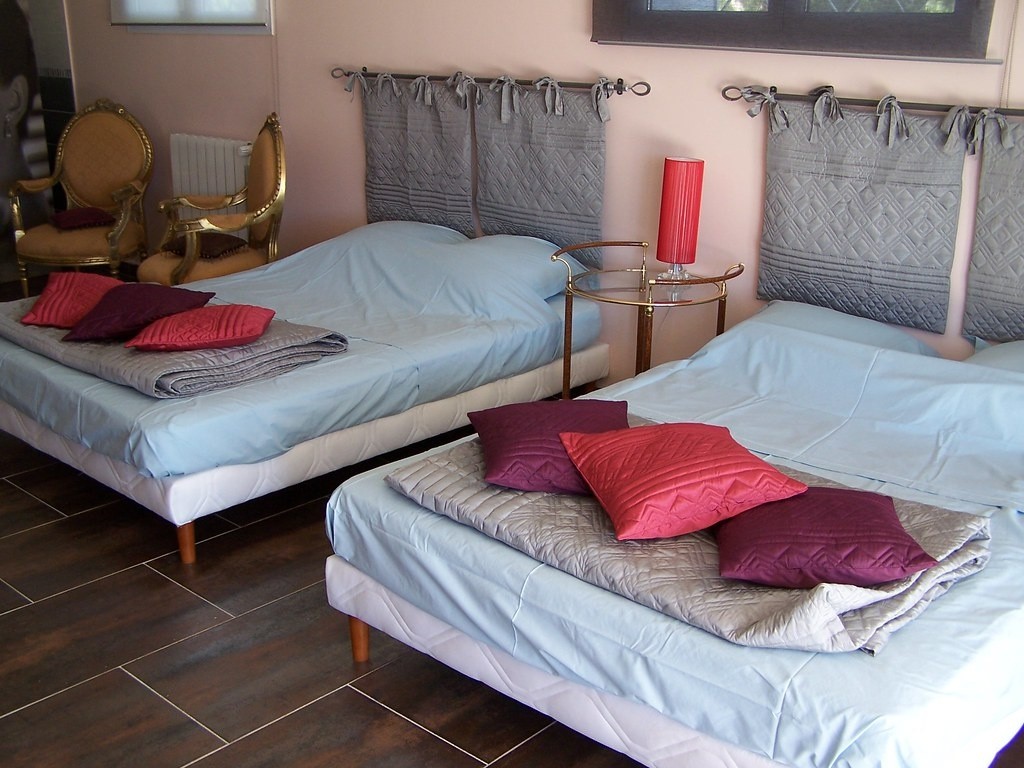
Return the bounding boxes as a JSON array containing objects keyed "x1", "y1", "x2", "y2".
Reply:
[
  {"x1": 327, "y1": 83, "x2": 1024, "y2": 768},
  {"x1": 0, "y1": 71, "x2": 653, "y2": 566}
]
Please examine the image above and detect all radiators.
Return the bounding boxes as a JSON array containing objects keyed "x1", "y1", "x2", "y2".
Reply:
[{"x1": 167, "y1": 132, "x2": 254, "y2": 243}]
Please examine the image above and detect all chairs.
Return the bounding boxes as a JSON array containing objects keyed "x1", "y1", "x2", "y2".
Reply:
[
  {"x1": 137, "y1": 113, "x2": 285, "y2": 285},
  {"x1": 10, "y1": 98, "x2": 156, "y2": 299}
]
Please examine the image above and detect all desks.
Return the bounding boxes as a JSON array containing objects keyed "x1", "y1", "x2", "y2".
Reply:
[{"x1": 549, "y1": 240, "x2": 746, "y2": 401}]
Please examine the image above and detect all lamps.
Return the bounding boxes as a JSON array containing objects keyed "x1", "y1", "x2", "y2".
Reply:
[{"x1": 655, "y1": 157, "x2": 705, "y2": 284}]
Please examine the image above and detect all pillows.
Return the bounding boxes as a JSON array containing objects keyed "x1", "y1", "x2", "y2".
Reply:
[
  {"x1": 50, "y1": 207, "x2": 116, "y2": 230},
  {"x1": 124, "y1": 304, "x2": 277, "y2": 351},
  {"x1": 465, "y1": 400, "x2": 627, "y2": 496},
  {"x1": 752, "y1": 298, "x2": 1024, "y2": 372},
  {"x1": 715, "y1": 486, "x2": 939, "y2": 587},
  {"x1": 163, "y1": 233, "x2": 248, "y2": 259},
  {"x1": 63, "y1": 283, "x2": 217, "y2": 341},
  {"x1": 354, "y1": 220, "x2": 591, "y2": 299},
  {"x1": 556, "y1": 421, "x2": 808, "y2": 541},
  {"x1": 20, "y1": 271, "x2": 124, "y2": 328}
]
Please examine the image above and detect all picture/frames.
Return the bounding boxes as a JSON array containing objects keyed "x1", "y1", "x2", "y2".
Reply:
[{"x1": 106, "y1": 0, "x2": 275, "y2": 35}]
